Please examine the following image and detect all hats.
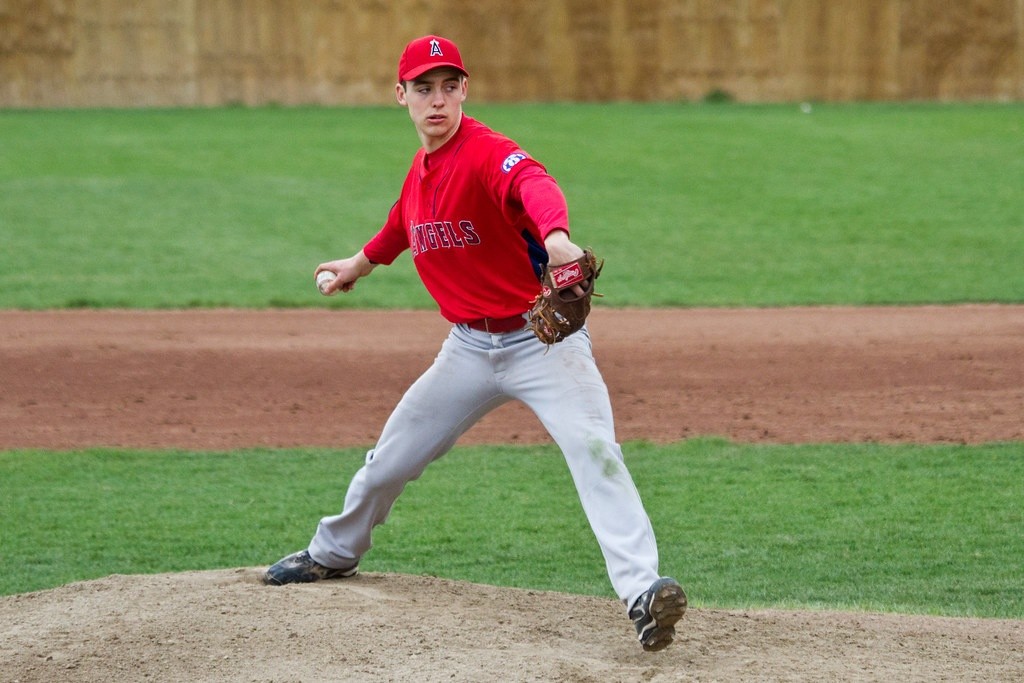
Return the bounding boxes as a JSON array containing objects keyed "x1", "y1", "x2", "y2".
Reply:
[{"x1": 397, "y1": 36, "x2": 469, "y2": 85}]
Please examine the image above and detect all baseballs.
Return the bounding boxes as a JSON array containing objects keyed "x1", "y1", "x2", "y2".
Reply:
[{"x1": 316, "y1": 270, "x2": 339, "y2": 296}]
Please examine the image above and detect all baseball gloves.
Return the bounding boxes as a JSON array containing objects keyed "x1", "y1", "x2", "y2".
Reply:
[{"x1": 528, "y1": 250, "x2": 597, "y2": 344}]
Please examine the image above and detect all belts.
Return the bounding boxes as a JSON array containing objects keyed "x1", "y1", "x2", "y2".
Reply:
[{"x1": 468, "y1": 315, "x2": 527, "y2": 334}]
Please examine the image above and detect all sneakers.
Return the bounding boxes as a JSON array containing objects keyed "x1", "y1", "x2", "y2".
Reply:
[
  {"x1": 264, "y1": 551, "x2": 358, "y2": 586},
  {"x1": 630, "y1": 576, "x2": 687, "y2": 652}
]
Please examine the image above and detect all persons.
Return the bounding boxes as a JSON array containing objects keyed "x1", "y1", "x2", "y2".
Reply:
[{"x1": 259, "y1": 34, "x2": 687, "y2": 652}]
